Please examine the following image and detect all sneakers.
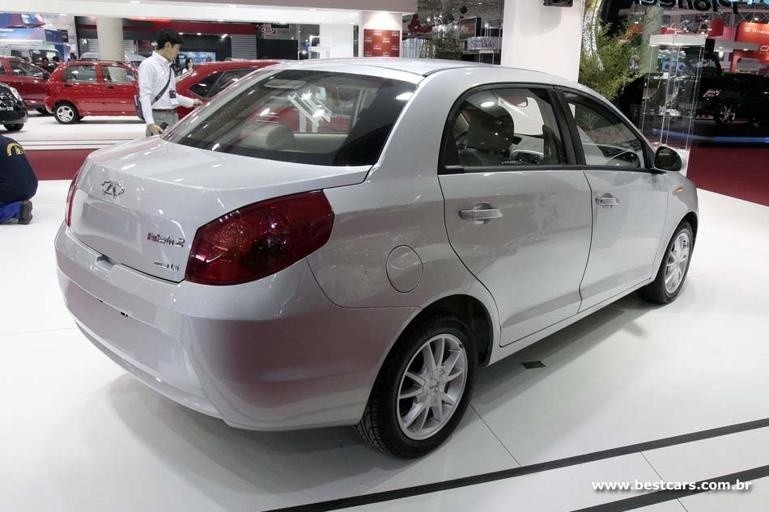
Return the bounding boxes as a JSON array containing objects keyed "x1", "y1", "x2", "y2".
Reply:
[{"x1": 19, "y1": 202, "x2": 33, "y2": 224}]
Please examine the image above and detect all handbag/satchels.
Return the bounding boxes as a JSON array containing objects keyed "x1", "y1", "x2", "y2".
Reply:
[{"x1": 136, "y1": 97, "x2": 146, "y2": 121}]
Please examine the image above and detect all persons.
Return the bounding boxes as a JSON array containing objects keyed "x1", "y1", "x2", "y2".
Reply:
[
  {"x1": 181, "y1": 58, "x2": 197, "y2": 75},
  {"x1": 1, "y1": 135, "x2": 38, "y2": 224},
  {"x1": 40, "y1": 57, "x2": 50, "y2": 72},
  {"x1": 134, "y1": 32, "x2": 205, "y2": 138},
  {"x1": 52, "y1": 57, "x2": 61, "y2": 68}
]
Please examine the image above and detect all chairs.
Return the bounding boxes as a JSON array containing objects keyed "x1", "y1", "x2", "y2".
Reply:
[
  {"x1": 459, "y1": 106, "x2": 513, "y2": 167},
  {"x1": 241, "y1": 120, "x2": 300, "y2": 152}
]
